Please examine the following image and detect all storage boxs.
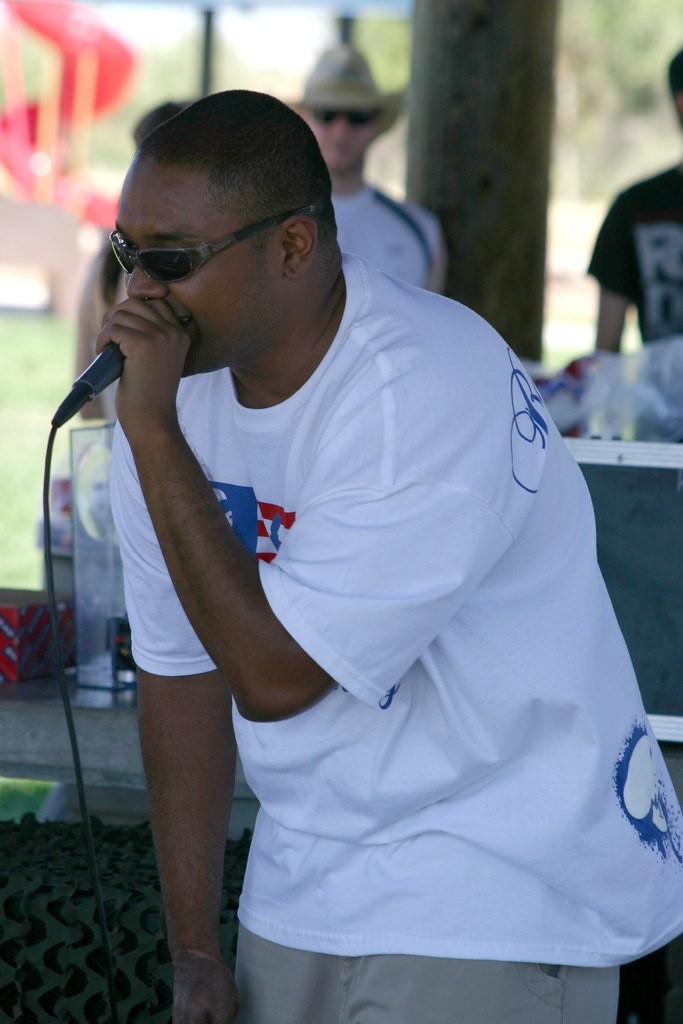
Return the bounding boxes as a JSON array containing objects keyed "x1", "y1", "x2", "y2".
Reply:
[
  {"x1": 0, "y1": 588, "x2": 76, "y2": 682},
  {"x1": 559, "y1": 435, "x2": 683, "y2": 744}
]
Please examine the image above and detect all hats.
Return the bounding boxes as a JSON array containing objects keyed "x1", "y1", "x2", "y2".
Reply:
[{"x1": 292, "y1": 41, "x2": 408, "y2": 143}]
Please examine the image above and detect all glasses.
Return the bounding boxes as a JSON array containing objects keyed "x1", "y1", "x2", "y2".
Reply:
[
  {"x1": 108, "y1": 203, "x2": 314, "y2": 287},
  {"x1": 308, "y1": 105, "x2": 379, "y2": 126}
]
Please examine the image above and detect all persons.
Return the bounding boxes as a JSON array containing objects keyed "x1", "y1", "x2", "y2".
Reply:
[
  {"x1": 279, "y1": 46, "x2": 448, "y2": 296},
  {"x1": 73, "y1": 101, "x2": 195, "y2": 466},
  {"x1": 96, "y1": 89, "x2": 683, "y2": 1024},
  {"x1": 588, "y1": 46, "x2": 683, "y2": 445}
]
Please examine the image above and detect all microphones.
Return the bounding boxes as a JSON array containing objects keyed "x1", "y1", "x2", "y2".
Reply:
[{"x1": 52, "y1": 342, "x2": 126, "y2": 428}]
